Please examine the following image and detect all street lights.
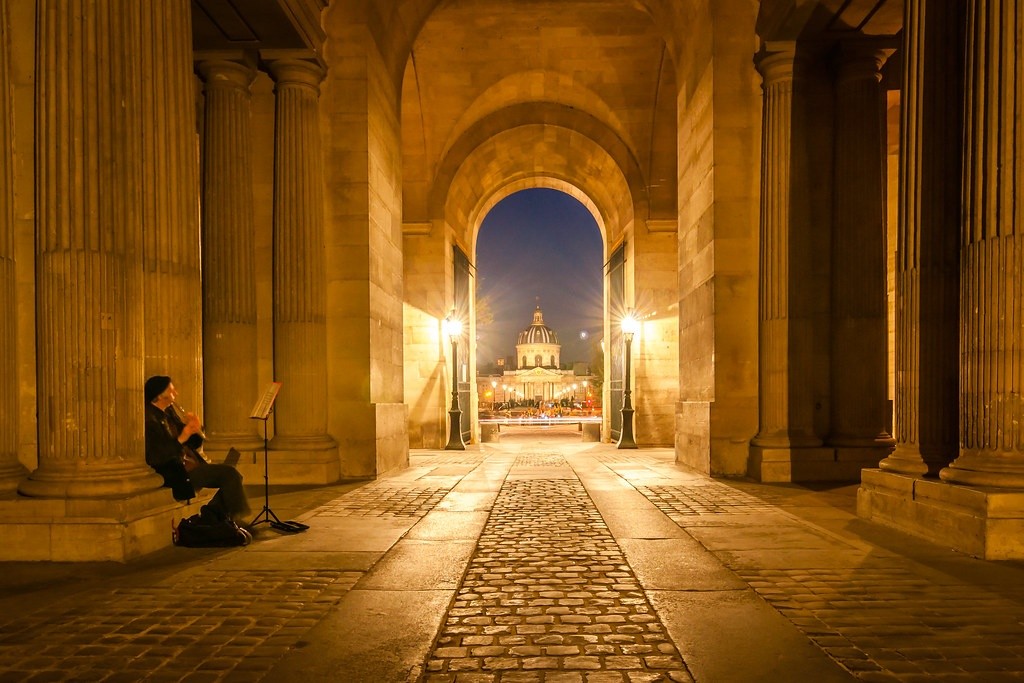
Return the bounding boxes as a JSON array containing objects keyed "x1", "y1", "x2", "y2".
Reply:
[
  {"x1": 572, "y1": 384, "x2": 576, "y2": 399},
  {"x1": 582, "y1": 381, "x2": 588, "y2": 401},
  {"x1": 502, "y1": 385, "x2": 507, "y2": 404},
  {"x1": 442, "y1": 318, "x2": 468, "y2": 451},
  {"x1": 508, "y1": 388, "x2": 512, "y2": 400},
  {"x1": 492, "y1": 381, "x2": 496, "y2": 410},
  {"x1": 617, "y1": 315, "x2": 641, "y2": 449}
]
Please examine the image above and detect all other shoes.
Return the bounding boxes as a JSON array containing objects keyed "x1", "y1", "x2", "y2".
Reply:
[
  {"x1": 233, "y1": 516, "x2": 257, "y2": 537},
  {"x1": 238, "y1": 527, "x2": 253, "y2": 545}
]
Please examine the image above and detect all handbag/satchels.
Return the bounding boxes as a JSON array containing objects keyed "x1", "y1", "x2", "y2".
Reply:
[{"x1": 175, "y1": 515, "x2": 246, "y2": 547}]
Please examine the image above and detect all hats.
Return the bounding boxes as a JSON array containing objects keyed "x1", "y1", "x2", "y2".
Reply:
[{"x1": 145, "y1": 376, "x2": 170, "y2": 403}]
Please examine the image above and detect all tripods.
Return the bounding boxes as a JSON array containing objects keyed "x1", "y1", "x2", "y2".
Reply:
[{"x1": 247, "y1": 397, "x2": 285, "y2": 530}]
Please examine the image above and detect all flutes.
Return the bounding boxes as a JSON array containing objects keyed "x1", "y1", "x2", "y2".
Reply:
[{"x1": 172, "y1": 401, "x2": 206, "y2": 440}]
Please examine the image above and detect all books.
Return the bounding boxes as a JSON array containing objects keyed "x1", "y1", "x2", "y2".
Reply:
[{"x1": 249, "y1": 382, "x2": 282, "y2": 417}]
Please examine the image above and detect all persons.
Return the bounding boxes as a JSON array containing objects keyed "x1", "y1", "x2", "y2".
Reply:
[
  {"x1": 552, "y1": 399, "x2": 560, "y2": 408},
  {"x1": 534, "y1": 400, "x2": 543, "y2": 413},
  {"x1": 144, "y1": 375, "x2": 252, "y2": 522},
  {"x1": 521, "y1": 409, "x2": 534, "y2": 419}
]
